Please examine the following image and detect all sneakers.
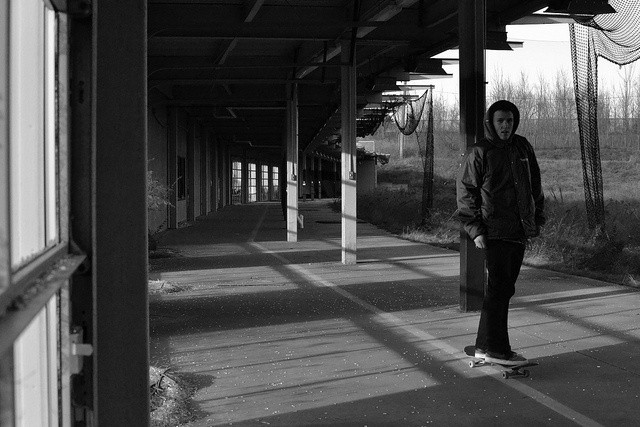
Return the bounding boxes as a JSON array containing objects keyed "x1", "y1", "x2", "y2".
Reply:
[
  {"x1": 474, "y1": 348, "x2": 490, "y2": 360},
  {"x1": 486, "y1": 352, "x2": 530, "y2": 365}
]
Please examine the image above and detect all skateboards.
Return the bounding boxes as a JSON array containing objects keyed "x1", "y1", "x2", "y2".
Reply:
[{"x1": 464, "y1": 343, "x2": 539, "y2": 379}]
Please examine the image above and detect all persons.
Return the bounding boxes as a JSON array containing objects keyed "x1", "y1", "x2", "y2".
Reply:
[{"x1": 457, "y1": 100, "x2": 546, "y2": 366}]
